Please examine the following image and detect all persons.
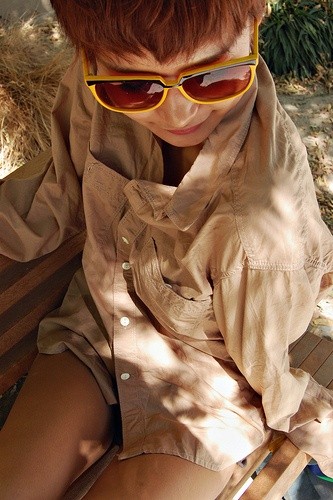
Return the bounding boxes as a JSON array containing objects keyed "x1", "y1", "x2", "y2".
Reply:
[{"x1": 0, "y1": 0, "x2": 333, "y2": 500}]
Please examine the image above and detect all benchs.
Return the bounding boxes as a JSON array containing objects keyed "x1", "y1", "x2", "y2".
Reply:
[{"x1": 0, "y1": 231, "x2": 333, "y2": 500}]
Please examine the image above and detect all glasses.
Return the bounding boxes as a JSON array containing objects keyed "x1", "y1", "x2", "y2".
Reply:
[{"x1": 81, "y1": 13, "x2": 259, "y2": 114}]
